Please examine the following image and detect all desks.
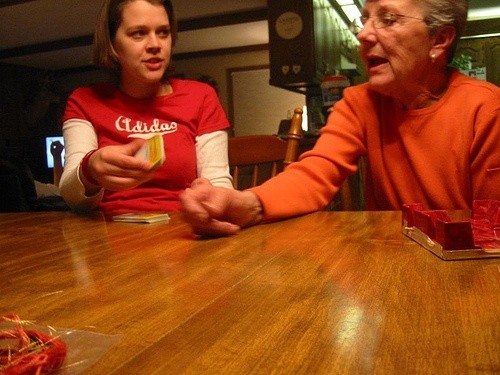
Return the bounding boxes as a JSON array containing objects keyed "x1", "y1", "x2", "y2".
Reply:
[{"x1": 1, "y1": 211, "x2": 500, "y2": 375}]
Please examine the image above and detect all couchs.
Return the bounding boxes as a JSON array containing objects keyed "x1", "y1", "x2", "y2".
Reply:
[{"x1": 0, "y1": 152, "x2": 71, "y2": 212}]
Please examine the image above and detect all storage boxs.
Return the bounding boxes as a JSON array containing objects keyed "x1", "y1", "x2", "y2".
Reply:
[{"x1": 320, "y1": 75, "x2": 351, "y2": 106}]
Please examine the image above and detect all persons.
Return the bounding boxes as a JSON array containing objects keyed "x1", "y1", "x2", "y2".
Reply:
[
  {"x1": 180, "y1": 0, "x2": 500, "y2": 234},
  {"x1": 59, "y1": 0, "x2": 234, "y2": 211}
]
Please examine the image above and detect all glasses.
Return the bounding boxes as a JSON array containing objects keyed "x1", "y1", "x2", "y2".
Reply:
[{"x1": 348, "y1": 11, "x2": 435, "y2": 33}]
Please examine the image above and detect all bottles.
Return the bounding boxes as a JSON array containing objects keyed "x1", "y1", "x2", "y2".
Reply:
[{"x1": 320, "y1": 75, "x2": 351, "y2": 107}]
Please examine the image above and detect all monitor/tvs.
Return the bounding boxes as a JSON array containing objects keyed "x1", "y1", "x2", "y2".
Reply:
[{"x1": 45, "y1": 135, "x2": 66, "y2": 169}]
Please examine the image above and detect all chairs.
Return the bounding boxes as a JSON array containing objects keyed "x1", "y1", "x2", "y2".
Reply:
[{"x1": 227, "y1": 108, "x2": 302, "y2": 191}]
